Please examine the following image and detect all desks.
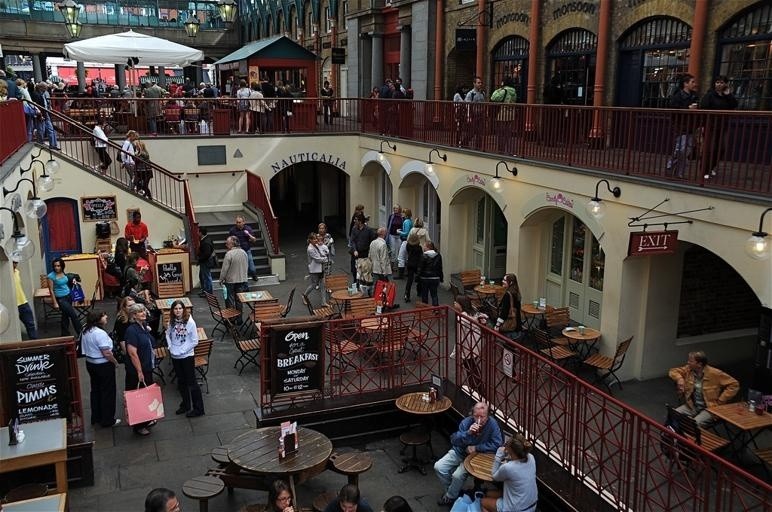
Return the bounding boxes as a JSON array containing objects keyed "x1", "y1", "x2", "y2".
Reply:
[
  {"x1": 395, "y1": 392, "x2": 452, "y2": 460},
  {"x1": 0, "y1": 418, "x2": 68, "y2": 493},
  {"x1": 1, "y1": 493, "x2": 67, "y2": 512},
  {"x1": 464, "y1": 452, "x2": 495, "y2": 494}
]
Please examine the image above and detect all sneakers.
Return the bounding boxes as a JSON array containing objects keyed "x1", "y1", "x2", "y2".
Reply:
[
  {"x1": 112, "y1": 418, "x2": 122, "y2": 428},
  {"x1": 438, "y1": 497, "x2": 455, "y2": 505},
  {"x1": 148, "y1": 420, "x2": 157, "y2": 427},
  {"x1": 134, "y1": 427, "x2": 153, "y2": 437}
]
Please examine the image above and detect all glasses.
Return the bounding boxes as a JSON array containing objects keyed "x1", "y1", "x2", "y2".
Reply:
[{"x1": 276, "y1": 497, "x2": 291, "y2": 503}]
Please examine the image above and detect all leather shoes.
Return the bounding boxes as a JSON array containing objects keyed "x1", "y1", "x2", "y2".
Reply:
[
  {"x1": 187, "y1": 408, "x2": 204, "y2": 417},
  {"x1": 321, "y1": 303, "x2": 331, "y2": 307},
  {"x1": 253, "y1": 275, "x2": 258, "y2": 281},
  {"x1": 176, "y1": 405, "x2": 191, "y2": 414},
  {"x1": 302, "y1": 296, "x2": 308, "y2": 305}
]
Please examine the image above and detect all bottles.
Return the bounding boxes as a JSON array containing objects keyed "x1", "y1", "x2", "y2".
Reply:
[
  {"x1": 278, "y1": 438, "x2": 285, "y2": 461},
  {"x1": 16, "y1": 430, "x2": 27, "y2": 442},
  {"x1": 284, "y1": 431, "x2": 291, "y2": 452},
  {"x1": 167, "y1": 231, "x2": 176, "y2": 241},
  {"x1": 343, "y1": 279, "x2": 368, "y2": 297},
  {"x1": 748, "y1": 397, "x2": 765, "y2": 416},
  {"x1": 221, "y1": 283, "x2": 228, "y2": 300},
  {"x1": 376, "y1": 285, "x2": 387, "y2": 312},
  {"x1": 289, "y1": 423, "x2": 296, "y2": 450},
  {"x1": 422, "y1": 386, "x2": 436, "y2": 405}
]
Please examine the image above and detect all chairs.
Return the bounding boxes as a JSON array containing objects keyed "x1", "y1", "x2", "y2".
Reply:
[
  {"x1": 35, "y1": 274, "x2": 100, "y2": 322},
  {"x1": 300, "y1": 271, "x2": 634, "y2": 396},
  {"x1": 658, "y1": 389, "x2": 772, "y2": 486},
  {"x1": 234, "y1": 287, "x2": 297, "y2": 376},
  {"x1": 151, "y1": 298, "x2": 214, "y2": 395},
  {"x1": 16, "y1": 83, "x2": 243, "y2": 139},
  {"x1": 202, "y1": 289, "x2": 241, "y2": 341},
  {"x1": 397, "y1": 430, "x2": 431, "y2": 476}
]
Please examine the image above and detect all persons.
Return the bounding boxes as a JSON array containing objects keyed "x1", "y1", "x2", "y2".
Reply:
[
  {"x1": 123, "y1": 303, "x2": 157, "y2": 434},
  {"x1": 369, "y1": 76, "x2": 410, "y2": 137},
  {"x1": 665, "y1": 74, "x2": 700, "y2": 179},
  {"x1": 324, "y1": 485, "x2": 374, "y2": 512},
  {"x1": 700, "y1": 76, "x2": 738, "y2": 180},
  {"x1": 453, "y1": 77, "x2": 518, "y2": 154},
  {"x1": 195, "y1": 216, "x2": 259, "y2": 327},
  {"x1": 114, "y1": 211, "x2": 161, "y2": 332},
  {"x1": 81, "y1": 308, "x2": 122, "y2": 429},
  {"x1": 321, "y1": 81, "x2": 334, "y2": 126},
  {"x1": 166, "y1": 300, "x2": 205, "y2": 417},
  {"x1": 455, "y1": 294, "x2": 482, "y2": 392},
  {"x1": 12, "y1": 261, "x2": 37, "y2": 341},
  {"x1": 46, "y1": 258, "x2": 83, "y2": 341},
  {"x1": 494, "y1": 273, "x2": 522, "y2": 381},
  {"x1": 300, "y1": 204, "x2": 443, "y2": 314},
  {"x1": 92, "y1": 118, "x2": 112, "y2": 174},
  {"x1": 121, "y1": 130, "x2": 153, "y2": 200},
  {"x1": 383, "y1": 496, "x2": 412, "y2": 512},
  {"x1": 434, "y1": 403, "x2": 502, "y2": 505},
  {"x1": 0, "y1": 68, "x2": 294, "y2": 152},
  {"x1": 669, "y1": 351, "x2": 741, "y2": 439},
  {"x1": 481, "y1": 434, "x2": 539, "y2": 512},
  {"x1": 145, "y1": 489, "x2": 179, "y2": 512},
  {"x1": 263, "y1": 480, "x2": 296, "y2": 512}
]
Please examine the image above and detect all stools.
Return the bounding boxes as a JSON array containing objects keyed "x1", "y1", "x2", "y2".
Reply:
[
  {"x1": 181, "y1": 425, "x2": 374, "y2": 512},
  {"x1": 4, "y1": 482, "x2": 49, "y2": 502}
]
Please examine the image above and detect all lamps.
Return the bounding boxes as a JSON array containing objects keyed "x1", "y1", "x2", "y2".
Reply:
[
  {"x1": 744, "y1": 205, "x2": 772, "y2": 261},
  {"x1": 489, "y1": 159, "x2": 519, "y2": 195},
  {"x1": 0, "y1": 145, "x2": 61, "y2": 266},
  {"x1": 586, "y1": 178, "x2": 622, "y2": 219},
  {"x1": 214, "y1": 0, "x2": 241, "y2": 25},
  {"x1": 373, "y1": 136, "x2": 397, "y2": 165},
  {"x1": 65, "y1": 21, "x2": 85, "y2": 39},
  {"x1": 58, "y1": 1, "x2": 81, "y2": 25},
  {"x1": 182, "y1": 7, "x2": 202, "y2": 39},
  {"x1": 422, "y1": 143, "x2": 447, "y2": 176}
]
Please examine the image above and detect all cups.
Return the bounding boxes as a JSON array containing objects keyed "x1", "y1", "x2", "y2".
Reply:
[
  {"x1": 243, "y1": 291, "x2": 265, "y2": 300},
  {"x1": 475, "y1": 418, "x2": 483, "y2": 435},
  {"x1": 532, "y1": 300, "x2": 539, "y2": 309},
  {"x1": 736, "y1": 402, "x2": 745, "y2": 414},
  {"x1": 578, "y1": 324, "x2": 585, "y2": 336},
  {"x1": 478, "y1": 275, "x2": 495, "y2": 289},
  {"x1": 133, "y1": 240, "x2": 140, "y2": 245}
]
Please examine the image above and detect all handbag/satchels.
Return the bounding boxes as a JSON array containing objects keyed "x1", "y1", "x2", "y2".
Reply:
[
  {"x1": 123, "y1": 381, "x2": 165, "y2": 426},
  {"x1": 374, "y1": 276, "x2": 397, "y2": 309},
  {"x1": 500, "y1": 290, "x2": 518, "y2": 333},
  {"x1": 74, "y1": 337, "x2": 86, "y2": 358},
  {"x1": 121, "y1": 265, "x2": 143, "y2": 296},
  {"x1": 109, "y1": 329, "x2": 129, "y2": 365},
  {"x1": 71, "y1": 284, "x2": 85, "y2": 301},
  {"x1": 204, "y1": 255, "x2": 218, "y2": 269}
]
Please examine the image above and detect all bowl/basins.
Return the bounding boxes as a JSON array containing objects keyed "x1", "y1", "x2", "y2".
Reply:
[{"x1": 164, "y1": 239, "x2": 174, "y2": 249}]
